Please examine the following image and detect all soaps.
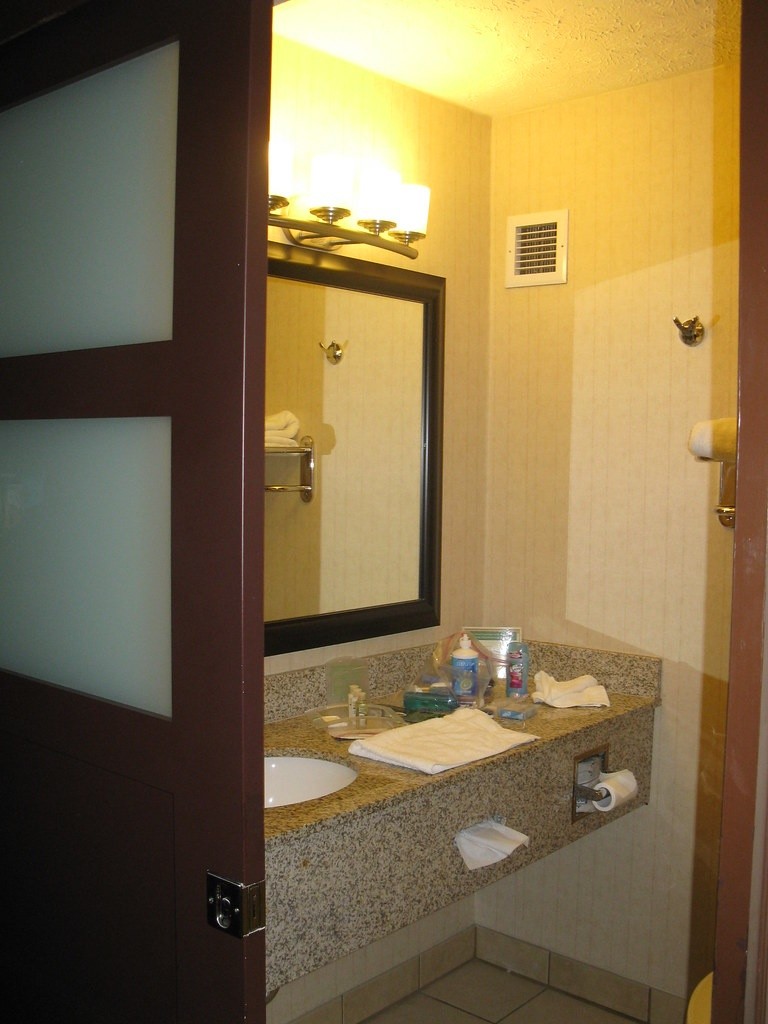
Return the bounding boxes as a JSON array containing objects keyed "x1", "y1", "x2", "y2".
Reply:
[{"x1": 429, "y1": 682, "x2": 453, "y2": 696}]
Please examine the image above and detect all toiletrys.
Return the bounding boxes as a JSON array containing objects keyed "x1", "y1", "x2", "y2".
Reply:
[
  {"x1": 347, "y1": 684, "x2": 369, "y2": 728},
  {"x1": 453, "y1": 633, "x2": 479, "y2": 696},
  {"x1": 506, "y1": 641, "x2": 529, "y2": 699}
]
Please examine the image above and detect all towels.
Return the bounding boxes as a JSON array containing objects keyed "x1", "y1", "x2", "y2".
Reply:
[
  {"x1": 349, "y1": 706, "x2": 542, "y2": 775},
  {"x1": 264, "y1": 409, "x2": 302, "y2": 449},
  {"x1": 531, "y1": 670, "x2": 611, "y2": 708},
  {"x1": 689, "y1": 418, "x2": 738, "y2": 465}
]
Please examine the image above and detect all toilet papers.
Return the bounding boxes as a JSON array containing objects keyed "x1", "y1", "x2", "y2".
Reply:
[{"x1": 591, "y1": 769, "x2": 639, "y2": 812}]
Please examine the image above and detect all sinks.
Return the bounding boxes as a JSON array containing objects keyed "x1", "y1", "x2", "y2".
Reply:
[{"x1": 265, "y1": 755, "x2": 358, "y2": 810}]
